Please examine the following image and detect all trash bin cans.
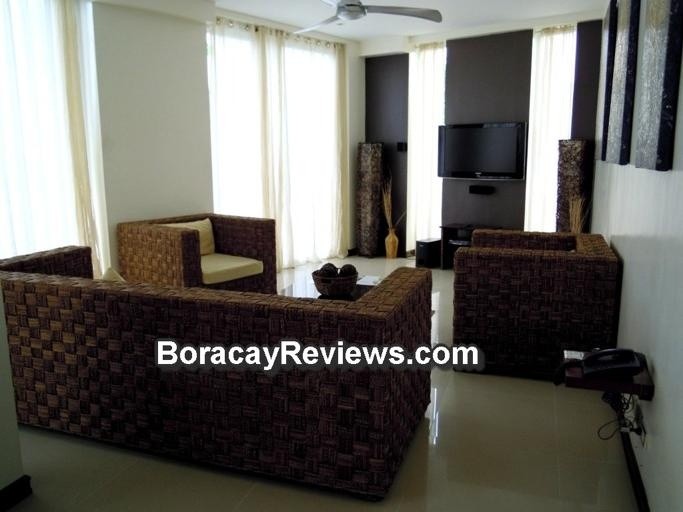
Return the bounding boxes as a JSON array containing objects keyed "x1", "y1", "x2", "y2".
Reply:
[{"x1": 449, "y1": 240, "x2": 471, "y2": 268}]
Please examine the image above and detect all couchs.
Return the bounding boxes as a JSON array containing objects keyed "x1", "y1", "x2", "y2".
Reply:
[{"x1": 0, "y1": 244, "x2": 433, "y2": 500}]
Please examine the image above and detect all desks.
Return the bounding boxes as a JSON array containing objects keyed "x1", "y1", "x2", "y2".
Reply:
[{"x1": 440, "y1": 223, "x2": 502, "y2": 270}]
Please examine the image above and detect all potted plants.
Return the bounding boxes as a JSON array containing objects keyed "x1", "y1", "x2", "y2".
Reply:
[{"x1": 381, "y1": 178, "x2": 407, "y2": 258}]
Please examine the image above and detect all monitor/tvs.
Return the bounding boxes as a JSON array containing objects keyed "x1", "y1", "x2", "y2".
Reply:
[{"x1": 438, "y1": 121, "x2": 528, "y2": 181}]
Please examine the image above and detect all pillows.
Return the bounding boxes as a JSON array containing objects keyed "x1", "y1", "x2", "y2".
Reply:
[{"x1": 155, "y1": 217, "x2": 215, "y2": 256}]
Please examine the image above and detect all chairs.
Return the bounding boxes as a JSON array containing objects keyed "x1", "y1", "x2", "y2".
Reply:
[
  {"x1": 451, "y1": 228, "x2": 623, "y2": 382},
  {"x1": 115, "y1": 212, "x2": 277, "y2": 295}
]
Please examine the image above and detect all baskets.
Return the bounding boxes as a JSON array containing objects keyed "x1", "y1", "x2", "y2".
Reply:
[{"x1": 312, "y1": 268, "x2": 358, "y2": 298}]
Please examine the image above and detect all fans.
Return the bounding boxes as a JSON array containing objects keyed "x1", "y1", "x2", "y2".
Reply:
[{"x1": 293, "y1": 0, "x2": 442, "y2": 35}]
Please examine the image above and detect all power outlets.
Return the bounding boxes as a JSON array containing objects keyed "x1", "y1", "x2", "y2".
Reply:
[{"x1": 637, "y1": 422, "x2": 646, "y2": 446}]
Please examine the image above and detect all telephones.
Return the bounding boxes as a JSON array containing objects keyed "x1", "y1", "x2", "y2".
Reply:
[{"x1": 581, "y1": 348, "x2": 641, "y2": 378}]
